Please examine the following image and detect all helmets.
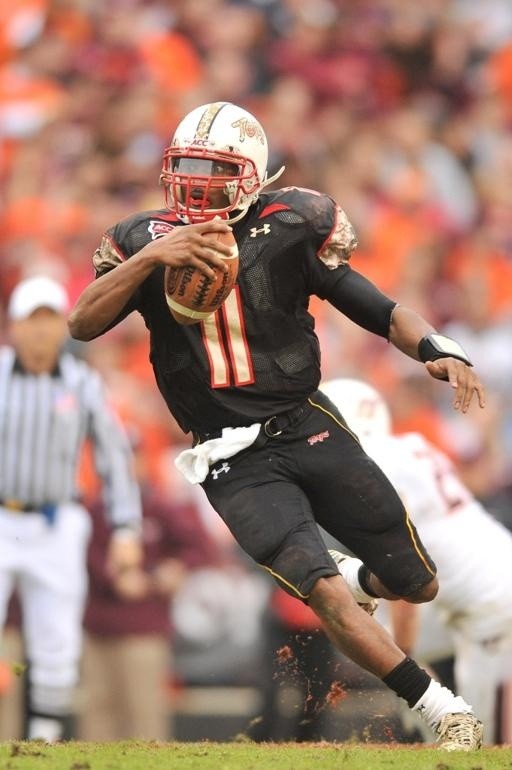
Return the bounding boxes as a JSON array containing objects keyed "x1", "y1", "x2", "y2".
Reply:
[{"x1": 150, "y1": 94, "x2": 278, "y2": 224}]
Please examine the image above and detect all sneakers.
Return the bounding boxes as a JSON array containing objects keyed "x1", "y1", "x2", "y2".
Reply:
[
  {"x1": 323, "y1": 549, "x2": 380, "y2": 624},
  {"x1": 435, "y1": 708, "x2": 483, "y2": 756}
]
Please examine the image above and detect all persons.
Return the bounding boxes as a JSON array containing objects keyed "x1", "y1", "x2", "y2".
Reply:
[
  {"x1": 0, "y1": 278, "x2": 141, "y2": 744},
  {"x1": 69, "y1": 101, "x2": 487, "y2": 752},
  {"x1": 318, "y1": 381, "x2": 511, "y2": 747},
  {"x1": 80, "y1": 429, "x2": 333, "y2": 742},
  {"x1": 0, "y1": 1, "x2": 511, "y2": 527}
]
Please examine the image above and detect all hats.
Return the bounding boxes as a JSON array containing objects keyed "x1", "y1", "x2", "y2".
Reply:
[{"x1": 9, "y1": 271, "x2": 67, "y2": 320}]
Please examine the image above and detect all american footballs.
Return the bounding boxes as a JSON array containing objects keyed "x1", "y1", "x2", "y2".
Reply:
[{"x1": 164, "y1": 225, "x2": 241, "y2": 327}]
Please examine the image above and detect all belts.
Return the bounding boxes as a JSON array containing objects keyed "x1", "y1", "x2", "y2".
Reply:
[{"x1": 191, "y1": 386, "x2": 328, "y2": 456}]
